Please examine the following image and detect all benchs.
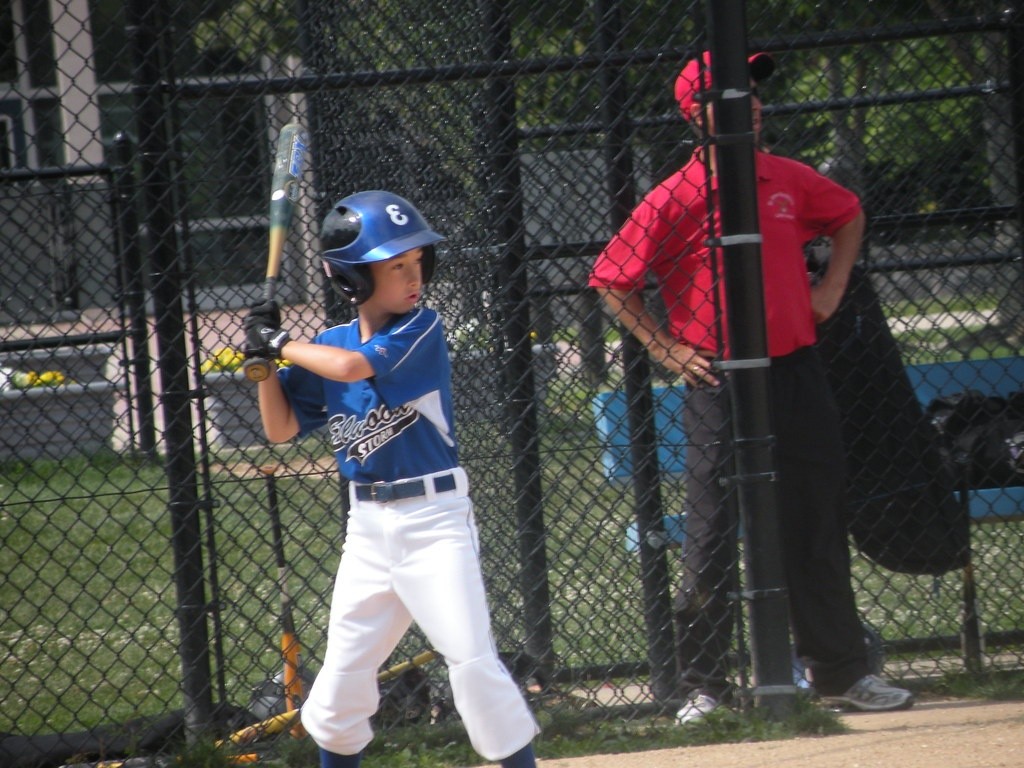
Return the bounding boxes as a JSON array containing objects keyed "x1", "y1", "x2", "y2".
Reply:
[{"x1": 592, "y1": 355, "x2": 1024, "y2": 673}]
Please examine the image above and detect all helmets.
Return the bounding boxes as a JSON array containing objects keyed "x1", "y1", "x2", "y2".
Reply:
[{"x1": 319, "y1": 190, "x2": 447, "y2": 305}]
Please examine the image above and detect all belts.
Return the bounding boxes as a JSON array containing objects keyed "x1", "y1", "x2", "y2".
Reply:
[{"x1": 356, "y1": 475, "x2": 456, "y2": 504}]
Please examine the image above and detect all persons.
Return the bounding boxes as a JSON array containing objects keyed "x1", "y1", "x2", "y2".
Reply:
[
  {"x1": 590, "y1": 48, "x2": 914, "y2": 723},
  {"x1": 241, "y1": 191, "x2": 545, "y2": 768}
]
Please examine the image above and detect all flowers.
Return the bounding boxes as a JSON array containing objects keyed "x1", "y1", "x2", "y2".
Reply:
[
  {"x1": 201, "y1": 347, "x2": 294, "y2": 374},
  {"x1": 0, "y1": 369, "x2": 77, "y2": 394},
  {"x1": 444, "y1": 319, "x2": 535, "y2": 352}
]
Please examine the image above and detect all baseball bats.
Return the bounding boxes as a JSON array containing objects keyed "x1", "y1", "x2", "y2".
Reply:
[
  {"x1": 257, "y1": 461, "x2": 311, "y2": 739},
  {"x1": 215, "y1": 649, "x2": 442, "y2": 751},
  {"x1": 241, "y1": 120, "x2": 312, "y2": 385},
  {"x1": 59, "y1": 752, "x2": 261, "y2": 768}
]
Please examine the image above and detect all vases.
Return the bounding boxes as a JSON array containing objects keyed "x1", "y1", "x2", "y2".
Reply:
[
  {"x1": 0, "y1": 381, "x2": 131, "y2": 462},
  {"x1": 447, "y1": 341, "x2": 562, "y2": 422},
  {"x1": 202, "y1": 368, "x2": 289, "y2": 449}
]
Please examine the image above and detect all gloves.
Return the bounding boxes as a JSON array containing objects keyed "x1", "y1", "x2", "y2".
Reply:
[
  {"x1": 245, "y1": 323, "x2": 292, "y2": 359},
  {"x1": 241, "y1": 298, "x2": 280, "y2": 330}
]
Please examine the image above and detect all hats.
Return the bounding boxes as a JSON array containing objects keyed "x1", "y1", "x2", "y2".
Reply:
[{"x1": 674, "y1": 51, "x2": 774, "y2": 125}]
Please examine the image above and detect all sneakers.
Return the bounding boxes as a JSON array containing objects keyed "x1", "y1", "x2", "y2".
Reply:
[
  {"x1": 819, "y1": 675, "x2": 913, "y2": 713},
  {"x1": 675, "y1": 692, "x2": 740, "y2": 730}
]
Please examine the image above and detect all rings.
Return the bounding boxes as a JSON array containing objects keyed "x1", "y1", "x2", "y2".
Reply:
[{"x1": 691, "y1": 365, "x2": 701, "y2": 373}]
